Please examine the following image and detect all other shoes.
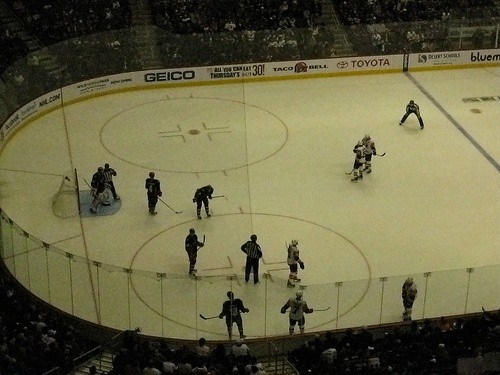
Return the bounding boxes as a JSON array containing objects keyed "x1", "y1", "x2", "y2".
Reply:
[
  {"x1": 114, "y1": 197, "x2": 120, "y2": 199},
  {"x1": 90, "y1": 208, "x2": 97, "y2": 214}
]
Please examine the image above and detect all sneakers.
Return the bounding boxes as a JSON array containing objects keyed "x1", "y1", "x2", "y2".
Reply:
[
  {"x1": 294, "y1": 276, "x2": 301, "y2": 283},
  {"x1": 363, "y1": 167, "x2": 371, "y2": 174},
  {"x1": 189, "y1": 269, "x2": 198, "y2": 279},
  {"x1": 352, "y1": 175, "x2": 363, "y2": 182},
  {"x1": 287, "y1": 279, "x2": 296, "y2": 287}
]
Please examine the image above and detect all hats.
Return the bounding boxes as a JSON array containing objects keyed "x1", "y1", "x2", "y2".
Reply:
[{"x1": 227, "y1": 291, "x2": 234, "y2": 297}]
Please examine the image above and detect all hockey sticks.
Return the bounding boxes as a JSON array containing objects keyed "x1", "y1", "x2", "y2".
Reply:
[
  {"x1": 188, "y1": 235, "x2": 206, "y2": 261},
  {"x1": 376, "y1": 152, "x2": 386, "y2": 157},
  {"x1": 199, "y1": 311, "x2": 242, "y2": 320},
  {"x1": 345, "y1": 169, "x2": 354, "y2": 175},
  {"x1": 193, "y1": 195, "x2": 228, "y2": 203},
  {"x1": 286, "y1": 240, "x2": 289, "y2": 249},
  {"x1": 158, "y1": 197, "x2": 184, "y2": 215},
  {"x1": 286, "y1": 306, "x2": 331, "y2": 313},
  {"x1": 82, "y1": 177, "x2": 95, "y2": 192}
]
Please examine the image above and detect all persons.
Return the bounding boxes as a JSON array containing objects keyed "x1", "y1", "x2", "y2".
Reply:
[
  {"x1": 287, "y1": 240, "x2": 305, "y2": 287},
  {"x1": 185, "y1": 228, "x2": 204, "y2": 276},
  {"x1": 101, "y1": 163, "x2": 120, "y2": 200},
  {"x1": 145, "y1": 172, "x2": 162, "y2": 214},
  {"x1": 351, "y1": 140, "x2": 366, "y2": 183},
  {"x1": 107, "y1": 309, "x2": 500, "y2": 375},
  {"x1": 0, "y1": 0, "x2": 500, "y2": 128},
  {"x1": 401, "y1": 277, "x2": 417, "y2": 320},
  {"x1": 0, "y1": 264, "x2": 99, "y2": 375},
  {"x1": 193, "y1": 184, "x2": 214, "y2": 219},
  {"x1": 399, "y1": 99, "x2": 424, "y2": 129},
  {"x1": 219, "y1": 291, "x2": 250, "y2": 339},
  {"x1": 241, "y1": 235, "x2": 262, "y2": 284},
  {"x1": 361, "y1": 134, "x2": 377, "y2": 173},
  {"x1": 89, "y1": 167, "x2": 111, "y2": 214},
  {"x1": 281, "y1": 291, "x2": 314, "y2": 334}
]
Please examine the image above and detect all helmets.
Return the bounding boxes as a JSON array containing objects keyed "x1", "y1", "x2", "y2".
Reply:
[
  {"x1": 207, "y1": 185, "x2": 212, "y2": 190},
  {"x1": 292, "y1": 239, "x2": 298, "y2": 245},
  {"x1": 296, "y1": 289, "x2": 302, "y2": 296},
  {"x1": 358, "y1": 140, "x2": 363, "y2": 146},
  {"x1": 410, "y1": 100, "x2": 414, "y2": 104},
  {"x1": 365, "y1": 134, "x2": 370, "y2": 140},
  {"x1": 405, "y1": 277, "x2": 414, "y2": 285},
  {"x1": 98, "y1": 167, "x2": 103, "y2": 170},
  {"x1": 105, "y1": 163, "x2": 109, "y2": 166},
  {"x1": 190, "y1": 228, "x2": 195, "y2": 232},
  {"x1": 149, "y1": 172, "x2": 155, "y2": 176},
  {"x1": 250, "y1": 234, "x2": 257, "y2": 240}
]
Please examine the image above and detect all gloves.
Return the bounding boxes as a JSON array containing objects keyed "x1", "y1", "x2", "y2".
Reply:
[
  {"x1": 245, "y1": 308, "x2": 249, "y2": 313},
  {"x1": 300, "y1": 261, "x2": 304, "y2": 270},
  {"x1": 309, "y1": 308, "x2": 313, "y2": 313},
  {"x1": 354, "y1": 144, "x2": 359, "y2": 149},
  {"x1": 281, "y1": 308, "x2": 286, "y2": 314},
  {"x1": 218, "y1": 315, "x2": 223, "y2": 319},
  {"x1": 373, "y1": 151, "x2": 376, "y2": 155}
]
